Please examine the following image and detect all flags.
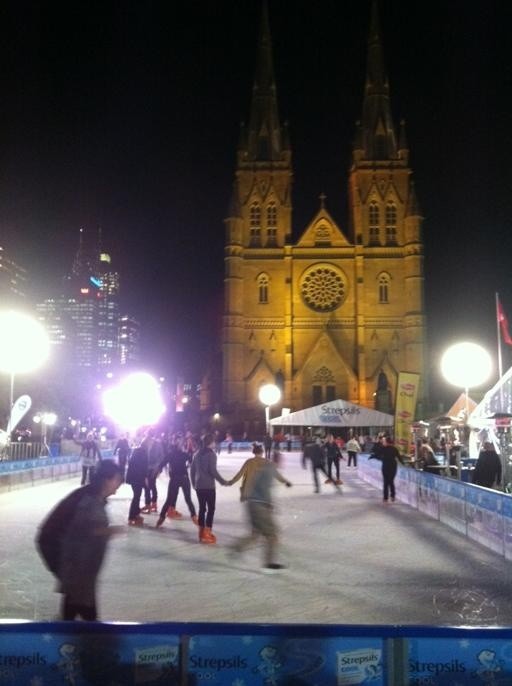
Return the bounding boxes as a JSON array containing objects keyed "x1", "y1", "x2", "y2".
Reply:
[{"x1": 496, "y1": 297, "x2": 511, "y2": 345}]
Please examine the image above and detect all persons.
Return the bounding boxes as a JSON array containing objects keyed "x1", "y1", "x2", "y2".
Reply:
[
  {"x1": 283, "y1": 431, "x2": 295, "y2": 450},
  {"x1": 273, "y1": 432, "x2": 287, "y2": 450},
  {"x1": 72, "y1": 434, "x2": 103, "y2": 488},
  {"x1": 228, "y1": 440, "x2": 294, "y2": 575},
  {"x1": 114, "y1": 428, "x2": 236, "y2": 544},
  {"x1": 296, "y1": 429, "x2": 447, "y2": 503},
  {"x1": 33, "y1": 459, "x2": 126, "y2": 622},
  {"x1": 13, "y1": 425, "x2": 35, "y2": 462},
  {"x1": 471, "y1": 438, "x2": 501, "y2": 489},
  {"x1": 263, "y1": 432, "x2": 272, "y2": 460}
]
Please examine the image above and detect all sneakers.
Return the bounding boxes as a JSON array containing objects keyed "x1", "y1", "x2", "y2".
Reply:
[
  {"x1": 128, "y1": 516, "x2": 144, "y2": 525},
  {"x1": 192, "y1": 515, "x2": 199, "y2": 525},
  {"x1": 151, "y1": 502, "x2": 157, "y2": 512},
  {"x1": 346, "y1": 466, "x2": 358, "y2": 470},
  {"x1": 141, "y1": 505, "x2": 151, "y2": 513},
  {"x1": 199, "y1": 527, "x2": 217, "y2": 543},
  {"x1": 260, "y1": 563, "x2": 289, "y2": 576},
  {"x1": 325, "y1": 479, "x2": 334, "y2": 484},
  {"x1": 334, "y1": 480, "x2": 343, "y2": 485},
  {"x1": 168, "y1": 506, "x2": 182, "y2": 518}
]
